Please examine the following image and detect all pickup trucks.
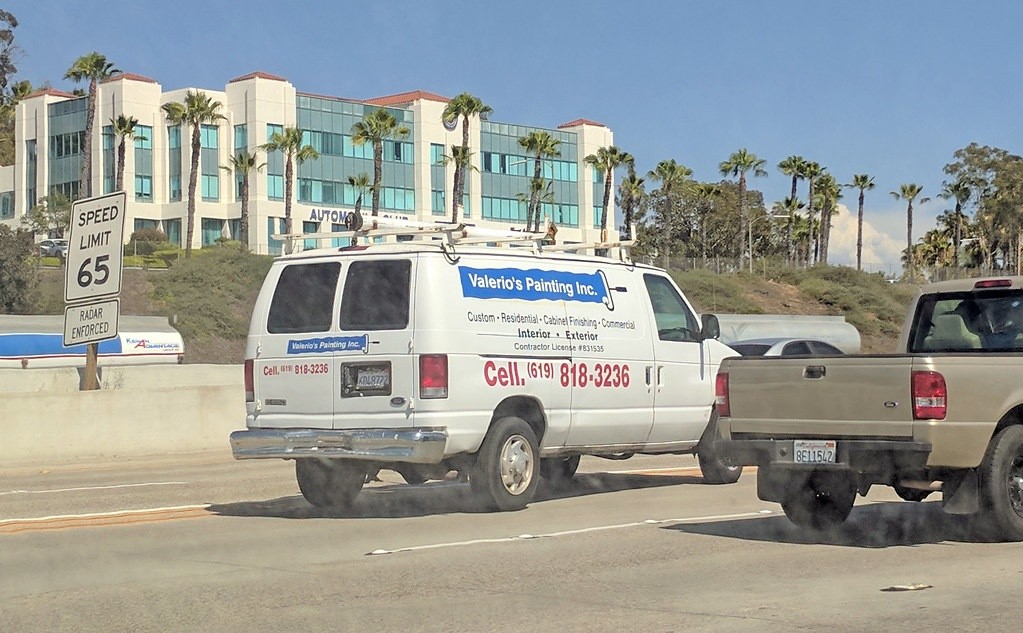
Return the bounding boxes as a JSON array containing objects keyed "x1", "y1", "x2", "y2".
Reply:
[{"x1": 715, "y1": 275, "x2": 1023, "y2": 542}]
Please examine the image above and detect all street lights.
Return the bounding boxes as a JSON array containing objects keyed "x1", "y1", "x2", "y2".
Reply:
[{"x1": 748, "y1": 214, "x2": 791, "y2": 273}]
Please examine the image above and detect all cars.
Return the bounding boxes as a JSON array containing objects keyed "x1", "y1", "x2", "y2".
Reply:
[{"x1": 39, "y1": 239, "x2": 68, "y2": 261}]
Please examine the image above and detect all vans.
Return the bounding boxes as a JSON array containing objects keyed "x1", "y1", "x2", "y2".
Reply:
[{"x1": 228, "y1": 211, "x2": 746, "y2": 512}]
